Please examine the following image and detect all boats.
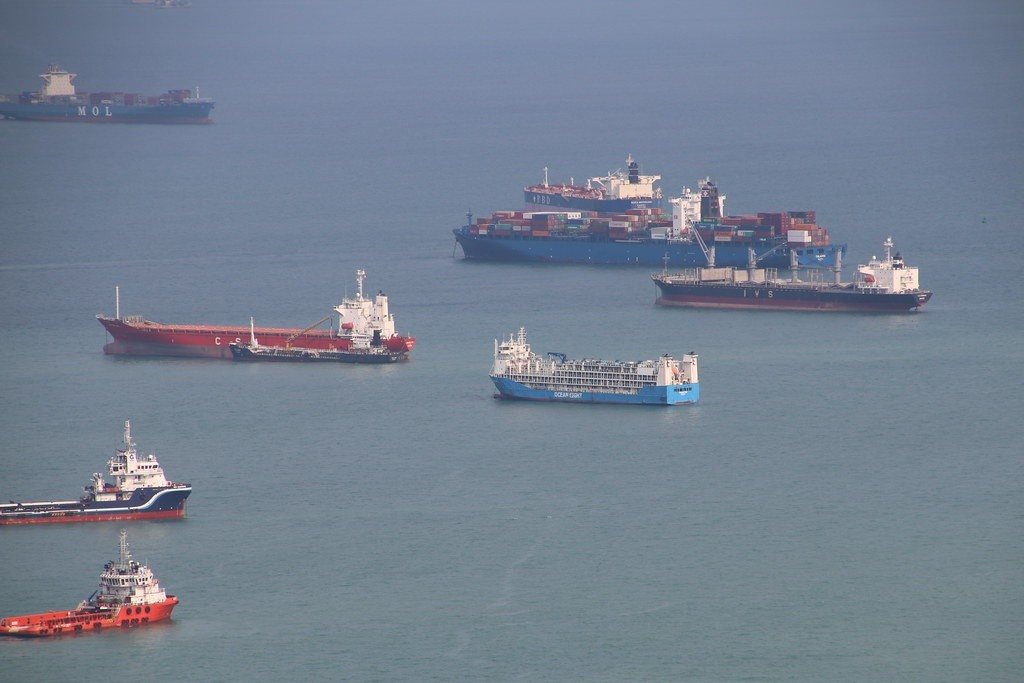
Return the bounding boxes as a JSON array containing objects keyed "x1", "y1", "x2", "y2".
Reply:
[
  {"x1": 451, "y1": 176, "x2": 848, "y2": 272},
  {"x1": 95, "y1": 270, "x2": 415, "y2": 360},
  {"x1": 1, "y1": 528, "x2": 179, "y2": 638},
  {"x1": 524, "y1": 154, "x2": 664, "y2": 214},
  {"x1": 489, "y1": 326, "x2": 700, "y2": 407},
  {"x1": 228, "y1": 315, "x2": 402, "y2": 362},
  {"x1": 0, "y1": 62, "x2": 217, "y2": 124},
  {"x1": 1, "y1": 420, "x2": 193, "y2": 521},
  {"x1": 651, "y1": 218, "x2": 933, "y2": 312}
]
